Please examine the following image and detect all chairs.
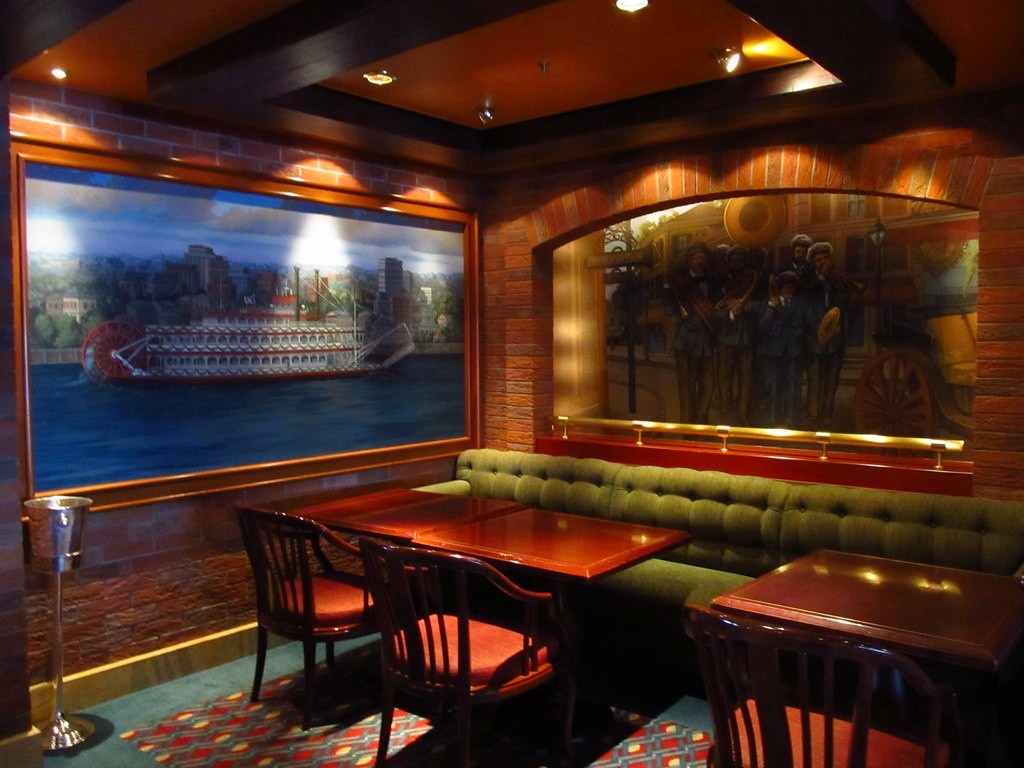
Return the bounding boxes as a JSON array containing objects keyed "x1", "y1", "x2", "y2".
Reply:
[
  {"x1": 361, "y1": 536, "x2": 580, "y2": 768},
  {"x1": 684, "y1": 602, "x2": 943, "y2": 767},
  {"x1": 233, "y1": 508, "x2": 379, "y2": 736}
]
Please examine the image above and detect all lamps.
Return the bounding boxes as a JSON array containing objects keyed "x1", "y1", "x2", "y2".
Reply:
[
  {"x1": 716, "y1": 47, "x2": 740, "y2": 73},
  {"x1": 362, "y1": 74, "x2": 393, "y2": 86},
  {"x1": 615, "y1": 0, "x2": 649, "y2": 12},
  {"x1": 52, "y1": 68, "x2": 67, "y2": 80}
]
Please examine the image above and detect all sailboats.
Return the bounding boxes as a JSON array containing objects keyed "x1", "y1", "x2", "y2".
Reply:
[{"x1": 108, "y1": 264, "x2": 417, "y2": 386}]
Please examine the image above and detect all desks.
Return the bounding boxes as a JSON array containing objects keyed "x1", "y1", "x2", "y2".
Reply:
[
  {"x1": 708, "y1": 547, "x2": 1024, "y2": 734},
  {"x1": 259, "y1": 486, "x2": 521, "y2": 663},
  {"x1": 410, "y1": 505, "x2": 693, "y2": 740}
]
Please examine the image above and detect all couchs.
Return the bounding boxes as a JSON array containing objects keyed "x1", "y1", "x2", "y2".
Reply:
[{"x1": 410, "y1": 448, "x2": 1024, "y2": 692}]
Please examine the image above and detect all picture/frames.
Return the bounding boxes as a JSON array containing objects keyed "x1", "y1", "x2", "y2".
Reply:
[
  {"x1": 6, "y1": 114, "x2": 484, "y2": 512},
  {"x1": 547, "y1": 192, "x2": 982, "y2": 454}
]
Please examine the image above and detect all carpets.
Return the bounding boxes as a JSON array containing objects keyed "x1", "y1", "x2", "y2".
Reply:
[{"x1": 119, "y1": 663, "x2": 720, "y2": 768}]
